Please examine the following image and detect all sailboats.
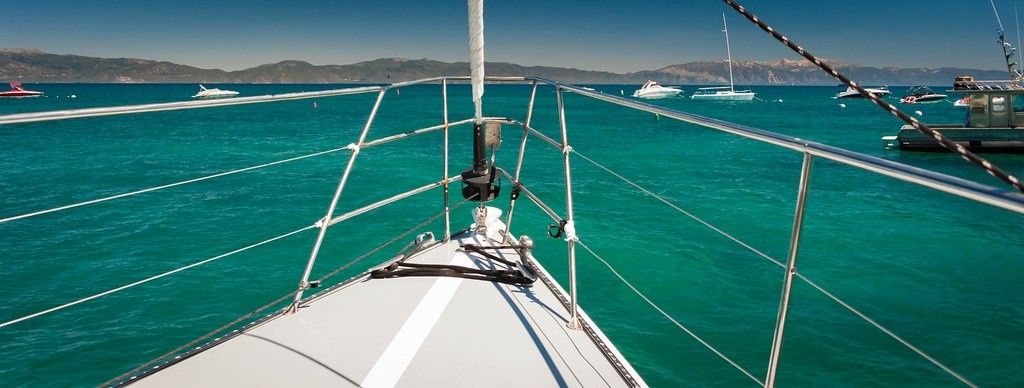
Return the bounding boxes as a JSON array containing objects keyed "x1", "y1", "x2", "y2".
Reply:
[{"x1": 690, "y1": 12, "x2": 755, "y2": 101}]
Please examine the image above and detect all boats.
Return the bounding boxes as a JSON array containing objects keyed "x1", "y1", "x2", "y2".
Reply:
[
  {"x1": 567, "y1": 86, "x2": 596, "y2": 92},
  {"x1": 897, "y1": 88, "x2": 1024, "y2": 153},
  {"x1": 945, "y1": 76, "x2": 1024, "y2": 96},
  {"x1": 952, "y1": 95, "x2": 1005, "y2": 112},
  {"x1": 191, "y1": 84, "x2": 240, "y2": 100},
  {"x1": 0, "y1": 82, "x2": 44, "y2": 97},
  {"x1": 835, "y1": 84, "x2": 890, "y2": 98},
  {"x1": 632, "y1": 80, "x2": 682, "y2": 99},
  {"x1": 900, "y1": 94, "x2": 948, "y2": 104}
]
